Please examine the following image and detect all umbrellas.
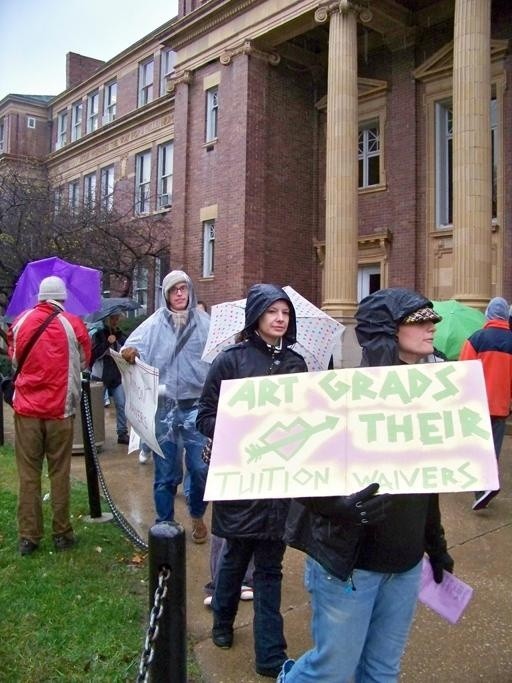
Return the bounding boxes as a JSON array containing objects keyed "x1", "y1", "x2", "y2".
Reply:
[
  {"x1": 86, "y1": 296, "x2": 141, "y2": 322},
  {"x1": 427, "y1": 298, "x2": 487, "y2": 361},
  {"x1": 201, "y1": 283, "x2": 346, "y2": 372},
  {"x1": 4, "y1": 256, "x2": 103, "y2": 318}
]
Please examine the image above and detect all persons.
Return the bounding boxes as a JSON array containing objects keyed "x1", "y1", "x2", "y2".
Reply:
[
  {"x1": 460, "y1": 296, "x2": 511, "y2": 511},
  {"x1": 194, "y1": 284, "x2": 307, "y2": 680},
  {"x1": 118, "y1": 269, "x2": 212, "y2": 545},
  {"x1": 277, "y1": 288, "x2": 457, "y2": 683},
  {"x1": 201, "y1": 528, "x2": 256, "y2": 611},
  {"x1": 6, "y1": 276, "x2": 92, "y2": 557},
  {"x1": 141, "y1": 297, "x2": 208, "y2": 496},
  {"x1": 88, "y1": 308, "x2": 131, "y2": 445}
]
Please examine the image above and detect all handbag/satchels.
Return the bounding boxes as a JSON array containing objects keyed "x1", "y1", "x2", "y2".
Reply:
[{"x1": 1, "y1": 379, "x2": 15, "y2": 405}]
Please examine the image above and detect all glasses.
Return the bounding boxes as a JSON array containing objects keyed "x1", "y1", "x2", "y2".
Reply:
[{"x1": 171, "y1": 285, "x2": 188, "y2": 294}]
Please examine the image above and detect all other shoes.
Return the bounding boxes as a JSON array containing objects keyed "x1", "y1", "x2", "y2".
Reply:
[
  {"x1": 204, "y1": 595, "x2": 211, "y2": 605},
  {"x1": 256, "y1": 661, "x2": 282, "y2": 678},
  {"x1": 192, "y1": 518, "x2": 208, "y2": 544},
  {"x1": 118, "y1": 433, "x2": 130, "y2": 446},
  {"x1": 139, "y1": 449, "x2": 151, "y2": 464},
  {"x1": 212, "y1": 632, "x2": 233, "y2": 649},
  {"x1": 106, "y1": 400, "x2": 110, "y2": 406},
  {"x1": 240, "y1": 585, "x2": 253, "y2": 601}
]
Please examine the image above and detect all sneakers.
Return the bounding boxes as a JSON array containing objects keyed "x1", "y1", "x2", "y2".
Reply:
[
  {"x1": 54, "y1": 534, "x2": 72, "y2": 551},
  {"x1": 473, "y1": 491, "x2": 500, "y2": 511},
  {"x1": 18, "y1": 539, "x2": 36, "y2": 555}
]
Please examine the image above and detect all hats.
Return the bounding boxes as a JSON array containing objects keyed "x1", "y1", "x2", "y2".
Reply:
[
  {"x1": 38, "y1": 276, "x2": 67, "y2": 301},
  {"x1": 401, "y1": 304, "x2": 443, "y2": 324}
]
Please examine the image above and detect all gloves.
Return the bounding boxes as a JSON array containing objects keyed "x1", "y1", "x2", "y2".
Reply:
[
  {"x1": 341, "y1": 483, "x2": 393, "y2": 529},
  {"x1": 429, "y1": 540, "x2": 454, "y2": 584}
]
